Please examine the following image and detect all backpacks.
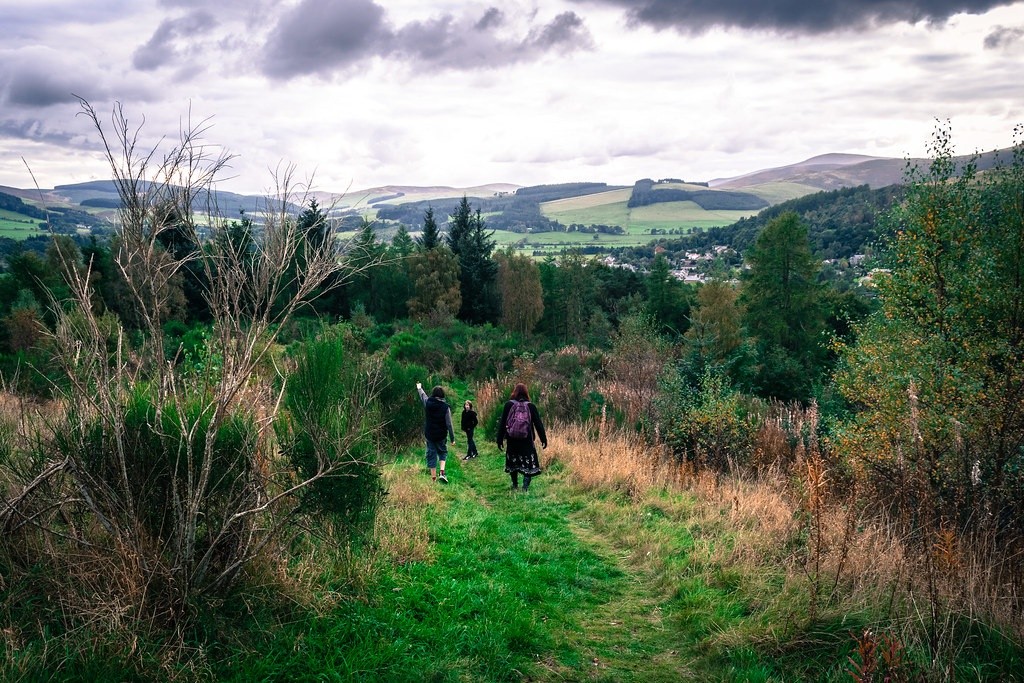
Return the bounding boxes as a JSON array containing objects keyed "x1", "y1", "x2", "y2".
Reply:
[{"x1": 506, "y1": 400, "x2": 531, "y2": 439}]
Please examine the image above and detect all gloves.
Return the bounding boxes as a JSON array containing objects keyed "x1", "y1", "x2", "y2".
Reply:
[
  {"x1": 542, "y1": 439, "x2": 547, "y2": 449},
  {"x1": 498, "y1": 442, "x2": 504, "y2": 451}
]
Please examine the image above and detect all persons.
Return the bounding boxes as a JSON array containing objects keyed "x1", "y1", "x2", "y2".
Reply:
[
  {"x1": 496, "y1": 383, "x2": 547, "y2": 490},
  {"x1": 460, "y1": 399, "x2": 479, "y2": 460},
  {"x1": 416, "y1": 382, "x2": 456, "y2": 484}
]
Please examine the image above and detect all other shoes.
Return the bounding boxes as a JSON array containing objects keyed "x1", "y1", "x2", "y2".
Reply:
[
  {"x1": 432, "y1": 477, "x2": 436, "y2": 482},
  {"x1": 439, "y1": 470, "x2": 448, "y2": 484},
  {"x1": 472, "y1": 453, "x2": 478, "y2": 457},
  {"x1": 464, "y1": 455, "x2": 472, "y2": 459}
]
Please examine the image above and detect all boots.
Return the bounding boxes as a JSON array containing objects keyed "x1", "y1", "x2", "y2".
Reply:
[
  {"x1": 513, "y1": 475, "x2": 518, "y2": 489},
  {"x1": 522, "y1": 477, "x2": 531, "y2": 492}
]
